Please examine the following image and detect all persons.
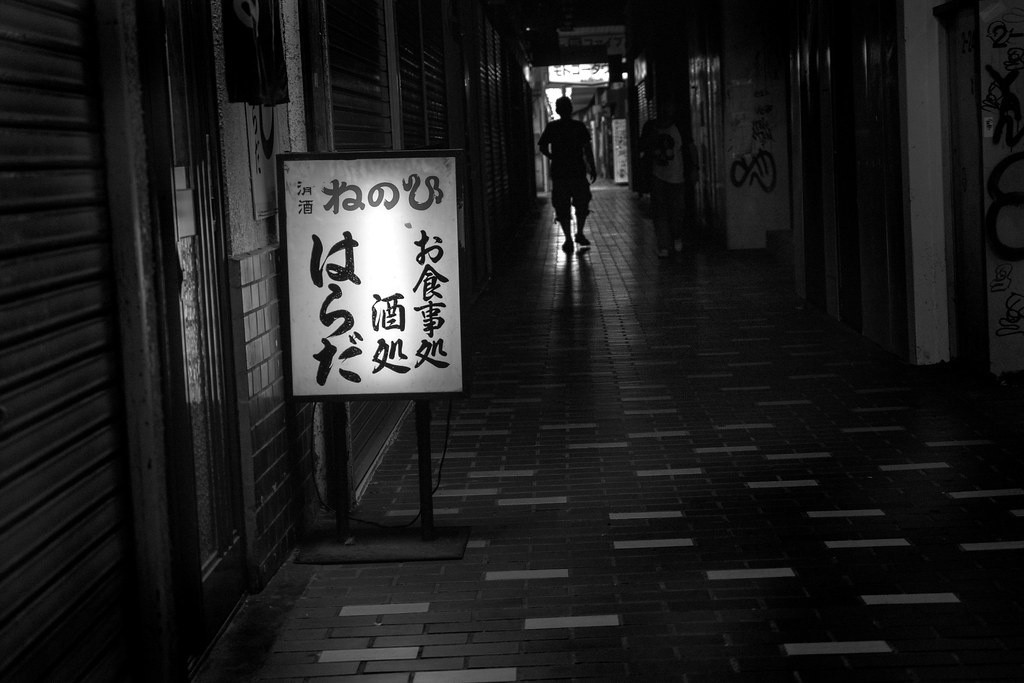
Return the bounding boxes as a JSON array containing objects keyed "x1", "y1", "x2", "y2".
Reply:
[
  {"x1": 640, "y1": 99, "x2": 694, "y2": 271},
  {"x1": 538, "y1": 96, "x2": 598, "y2": 252}
]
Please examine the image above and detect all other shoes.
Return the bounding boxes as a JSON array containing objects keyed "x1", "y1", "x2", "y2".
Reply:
[
  {"x1": 574, "y1": 232, "x2": 591, "y2": 245},
  {"x1": 562, "y1": 239, "x2": 574, "y2": 254}
]
